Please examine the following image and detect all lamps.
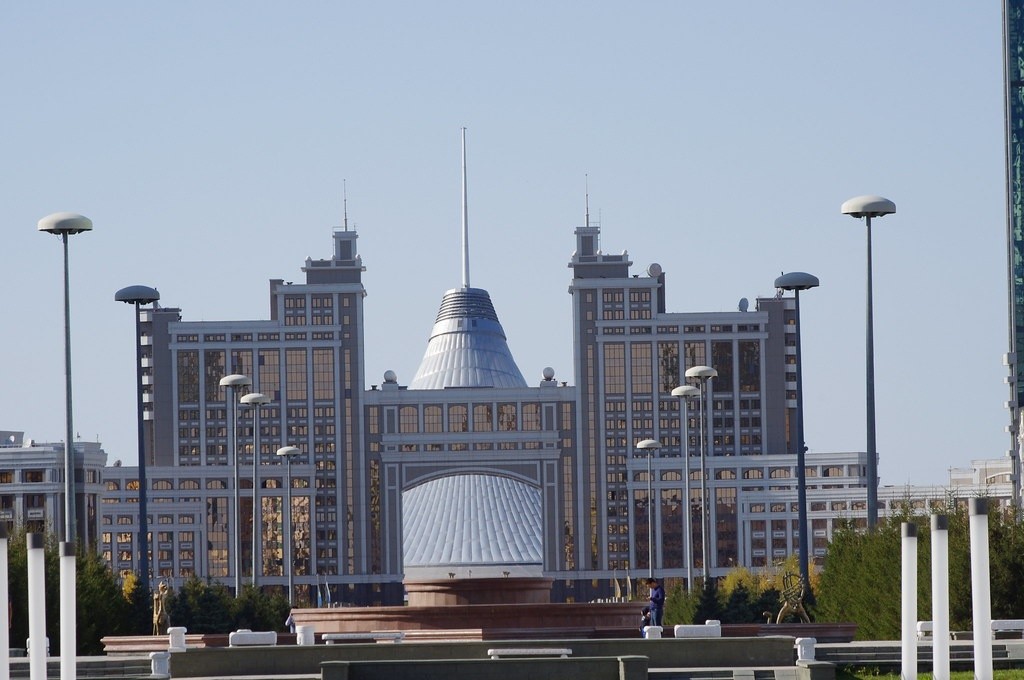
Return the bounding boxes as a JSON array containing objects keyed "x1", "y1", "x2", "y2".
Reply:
[
  {"x1": 503, "y1": 571, "x2": 510, "y2": 578},
  {"x1": 286, "y1": 281, "x2": 293, "y2": 285},
  {"x1": 177, "y1": 314, "x2": 182, "y2": 323},
  {"x1": 449, "y1": 573, "x2": 456, "y2": 578},
  {"x1": 371, "y1": 385, "x2": 377, "y2": 390},
  {"x1": 633, "y1": 275, "x2": 639, "y2": 278},
  {"x1": 561, "y1": 381, "x2": 568, "y2": 387}
]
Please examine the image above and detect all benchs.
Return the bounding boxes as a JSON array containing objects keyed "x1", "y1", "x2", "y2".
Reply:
[
  {"x1": 228, "y1": 631, "x2": 278, "y2": 647},
  {"x1": 487, "y1": 648, "x2": 572, "y2": 658},
  {"x1": 674, "y1": 625, "x2": 722, "y2": 638},
  {"x1": 322, "y1": 633, "x2": 405, "y2": 644}
]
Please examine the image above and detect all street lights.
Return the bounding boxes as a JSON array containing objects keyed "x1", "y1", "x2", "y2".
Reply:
[
  {"x1": 35, "y1": 211, "x2": 93, "y2": 543},
  {"x1": 217, "y1": 373, "x2": 270, "y2": 598},
  {"x1": 840, "y1": 192, "x2": 896, "y2": 538},
  {"x1": 635, "y1": 439, "x2": 663, "y2": 599},
  {"x1": 114, "y1": 285, "x2": 161, "y2": 599},
  {"x1": 775, "y1": 271, "x2": 819, "y2": 591},
  {"x1": 275, "y1": 445, "x2": 303, "y2": 633},
  {"x1": 671, "y1": 365, "x2": 719, "y2": 595}
]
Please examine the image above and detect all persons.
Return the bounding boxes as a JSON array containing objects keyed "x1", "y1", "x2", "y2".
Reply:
[
  {"x1": 640, "y1": 608, "x2": 650, "y2": 638},
  {"x1": 646, "y1": 578, "x2": 665, "y2": 626}
]
